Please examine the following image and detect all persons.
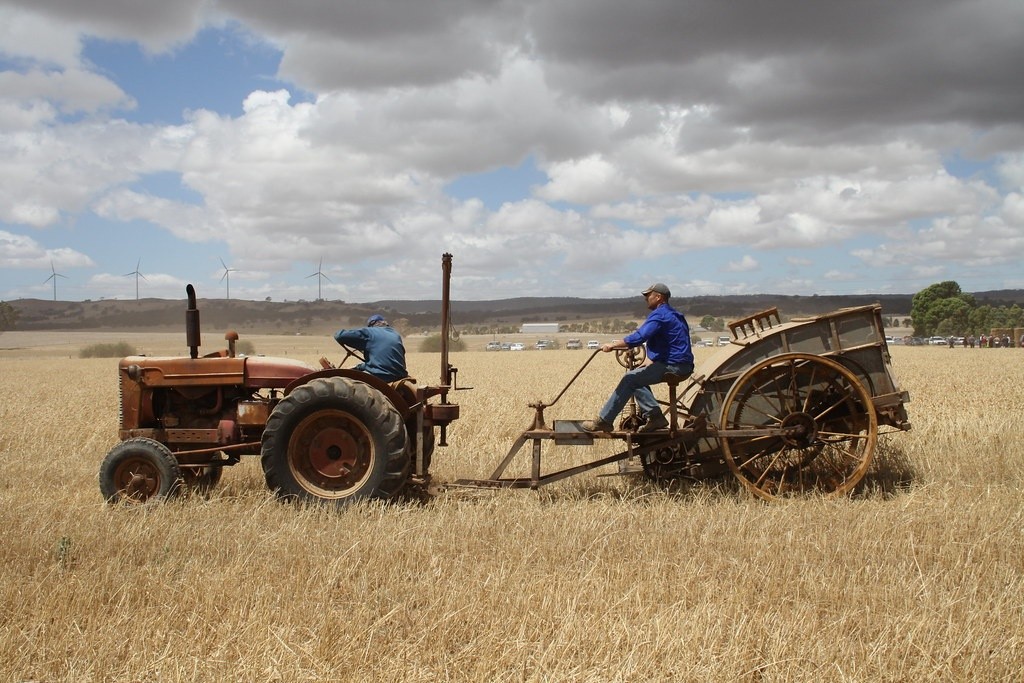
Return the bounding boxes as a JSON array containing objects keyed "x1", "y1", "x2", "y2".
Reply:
[
  {"x1": 334, "y1": 314, "x2": 408, "y2": 383},
  {"x1": 947, "y1": 334, "x2": 1015, "y2": 348},
  {"x1": 580, "y1": 283, "x2": 694, "y2": 433}
]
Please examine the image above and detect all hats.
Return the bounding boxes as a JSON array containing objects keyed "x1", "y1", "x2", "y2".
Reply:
[
  {"x1": 641, "y1": 283, "x2": 671, "y2": 298},
  {"x1": 367, "y1": 314, "x2": 384, "y2": 326}
]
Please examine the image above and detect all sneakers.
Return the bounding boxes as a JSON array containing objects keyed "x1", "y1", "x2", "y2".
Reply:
[
  {"x1": 639, "y1": 413, "x2": 669, "y2": 432},
  {"x1": 582, "y1": 416, "x2": 613, "y2": 432}
]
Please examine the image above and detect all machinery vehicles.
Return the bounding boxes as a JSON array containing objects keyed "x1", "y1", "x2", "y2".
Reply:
[{"x1": 99, "y1": 251, "x2": 911, "y2": 503}]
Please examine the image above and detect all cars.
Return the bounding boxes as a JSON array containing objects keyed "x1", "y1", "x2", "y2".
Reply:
[
  {"x1": 535, "y1": 339, "x2": 559, "y2": 350},
  {"x1": 885, "y1": 336, "x2": 964, "y2": 346},
  {"x1": 566, "y1": 338, "x2": 583, "y2": 350},
  {"x1": 586, "y1": 341, "x2": 600, "y2": 350},
  {"x1": 486, "y1": 340, "x2": 526, "y2": 351},
  {"x1": 696, "y1": 336, "x2": 730, "y2": 348}
]
